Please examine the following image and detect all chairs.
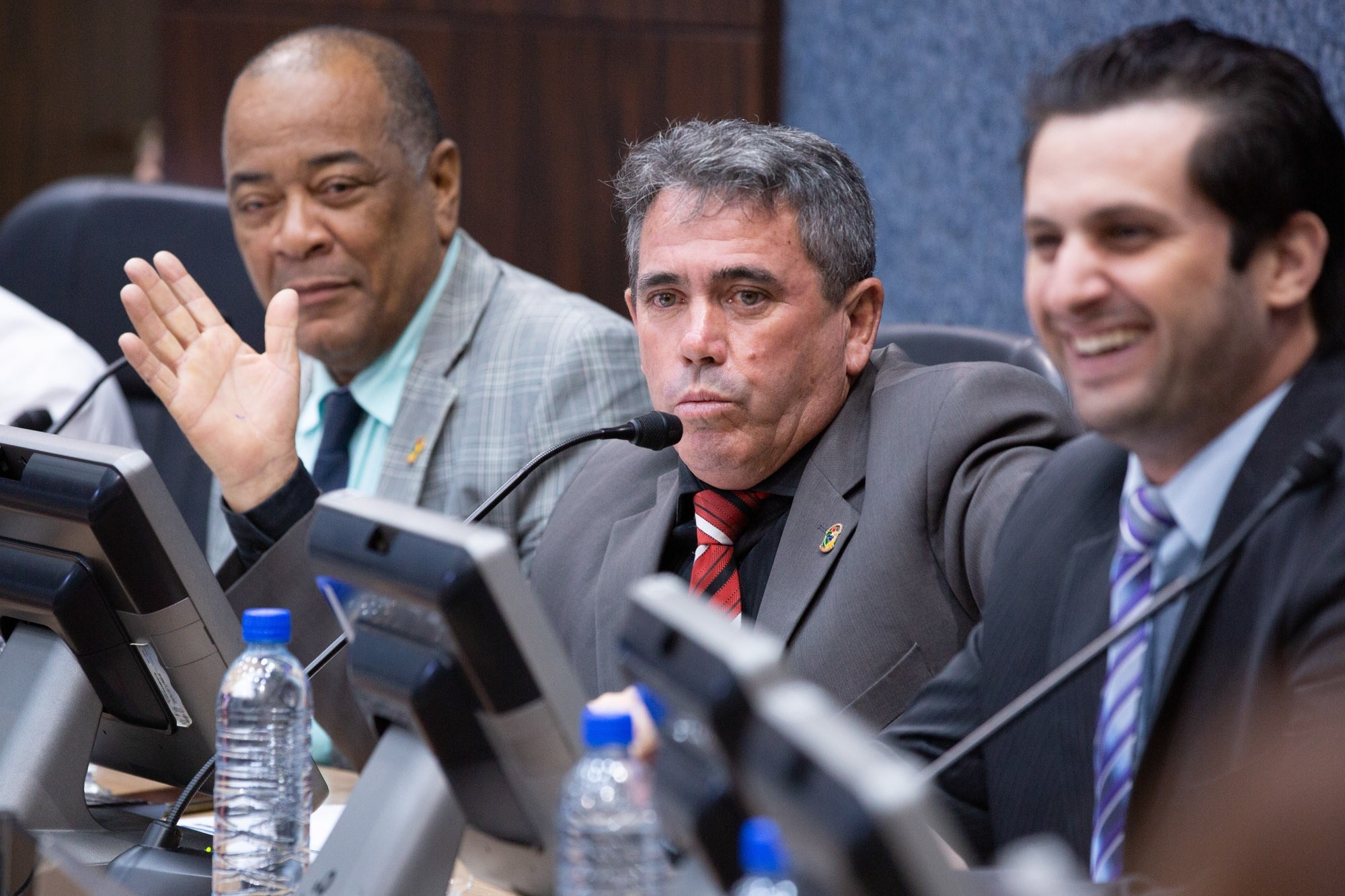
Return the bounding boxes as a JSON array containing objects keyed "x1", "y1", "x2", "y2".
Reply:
[{"x1": 0, "y1": 176, "x2": 269, "y2": 384}]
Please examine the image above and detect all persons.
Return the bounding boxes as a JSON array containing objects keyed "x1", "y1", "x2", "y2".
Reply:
[
  {"x1": 870, "y1": 17, "x2": 1344, "y2": 895},
  {"x1": 203, "y1": 30, "x2": 654, "y2": 771},
  {"x1": 0, "y1": 284, "x2": 142, "y2": 449},
  {"x1": 117, "y1": 118, "x2": 1078, "y2": 896}
]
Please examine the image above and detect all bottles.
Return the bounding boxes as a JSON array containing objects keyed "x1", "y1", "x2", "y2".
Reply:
[
  {"x1": 559, "y1": 712, "x2": 665, "y2": 896},
  {"x1": 211, "y1": 609, "x2": 315, "y2": 896},
  {"x1": 729, "y1": 817, "x2": 807, "y2": 896}
]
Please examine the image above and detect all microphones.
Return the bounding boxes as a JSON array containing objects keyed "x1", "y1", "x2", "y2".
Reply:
[
  {"x1": 141, "y1": 410, "x2": 683, "y2": 849},
  {"x1": 917, "y1": 431, "x2": 1345, "y2": 789},
  {"x1": 12, "y1": 410, "x2": 53, "y2": 438}
]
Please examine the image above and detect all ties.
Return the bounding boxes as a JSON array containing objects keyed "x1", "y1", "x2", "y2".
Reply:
[
  {"x1": 312, "y1": 389, "x2": 366, "y2": 496},
  {"x1": 1085, "y1": 485, "x2": 1177, "y2": 882},
  {"x1": 688, "y1": 487, "x2": 764, "y2": 632}
]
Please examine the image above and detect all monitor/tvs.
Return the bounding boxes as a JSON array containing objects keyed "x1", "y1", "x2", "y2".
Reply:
[
  {"x1": 620, "y1": 568, "x2": 978, "y2": 896},
  {"x1": 286, "y1": 487, "x2": 589, "y2": 896},
  {"x1": 0, "y1": 424, "x2": 330, "y2": 896}
]
